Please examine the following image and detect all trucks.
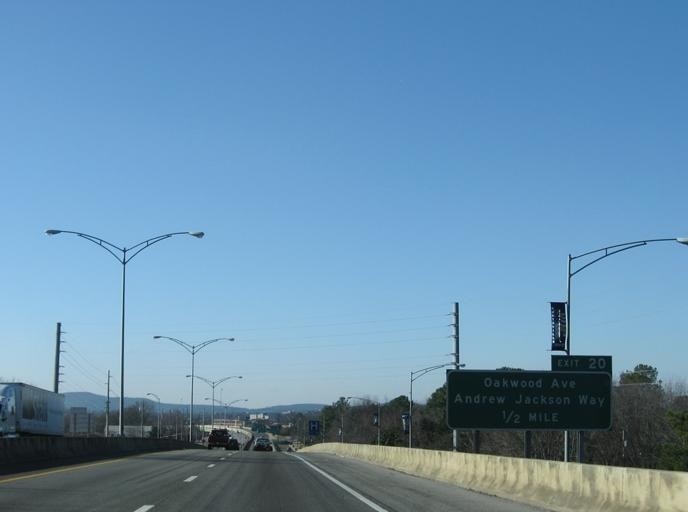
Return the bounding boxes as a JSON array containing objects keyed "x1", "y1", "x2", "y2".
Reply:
[{"x1": 0, "y1": 382, "x2": 67, "y2": 442}]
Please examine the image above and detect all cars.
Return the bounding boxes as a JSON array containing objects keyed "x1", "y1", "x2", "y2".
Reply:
[
  {"x1": 252, "y1": 436, "x2": 273, "y2": 451},
  {"x1": 225, "y1": 438, "x2": 240, "y2": 451}
]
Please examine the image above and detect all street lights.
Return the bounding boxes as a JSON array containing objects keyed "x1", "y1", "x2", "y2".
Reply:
[
  {"x1": 204, "y1": 396, "x2": 248, "y2": 429},
  {"x1": 339, "y1": 396, "x2": 381, "y2": 446},
  {"x1": 44, "y1": 225, "x2": 205, "y2": 438},
  {"x1": 152, "y1": 334, "x2": 235, "y2": 445},
  {"x1": 560, "y1": 236, "x2": 687, "y2": 464},
  {"x1": 406, "y1": 362, "x2": 466, "y2": 449},
  {"x1": 144, "y1": 392, "x2": 163, "y2": 440},
  {"x1": 185, "y1": 374, "x2": 243, "y2": 431}
]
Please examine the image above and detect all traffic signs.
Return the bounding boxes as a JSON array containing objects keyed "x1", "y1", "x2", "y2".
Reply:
[{"x1": 443, "y1": 368, "x2": 612, "y2": 432}]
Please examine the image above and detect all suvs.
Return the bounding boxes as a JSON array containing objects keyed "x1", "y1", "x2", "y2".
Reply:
[{"x1": 205, "y1": 427, "x2": 233, "y2": 450}]
